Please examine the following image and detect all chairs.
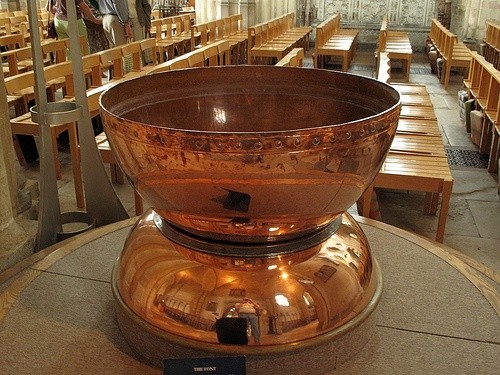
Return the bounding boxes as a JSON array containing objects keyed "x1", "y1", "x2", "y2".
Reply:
[{"x1": 0, "y1": 6, "x2": 500, "y2": 245}]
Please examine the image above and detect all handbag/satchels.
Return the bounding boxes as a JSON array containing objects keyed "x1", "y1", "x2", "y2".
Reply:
[
  {"x1": 46, "y1": 21, "x2": 57, "y2": 40},
  {"x1": 143, "y1": 32, "x2": 158, "y2": 62},
  {"x1": 122, "y1": 38, "x2": 143, "y2": 75}
]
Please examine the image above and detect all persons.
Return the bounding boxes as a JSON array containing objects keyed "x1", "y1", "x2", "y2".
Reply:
[
  {"x1": 235, "y1": 298, "x2": 260, "y2": 345},
  {"x1": 46, "y1": 0, "x2": 153, "y2": 77}
]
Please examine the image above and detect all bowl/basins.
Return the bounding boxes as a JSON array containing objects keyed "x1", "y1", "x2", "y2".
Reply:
[
  {"x1": 98, "y1": 64, "x2": 401, "y2": 241},
  {"x1": 108, "y1": 208, "x2": 386, "y2": 354}
]
